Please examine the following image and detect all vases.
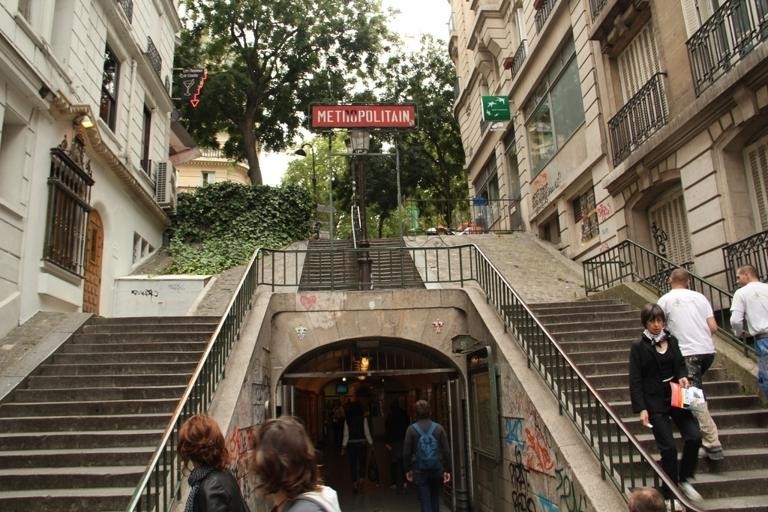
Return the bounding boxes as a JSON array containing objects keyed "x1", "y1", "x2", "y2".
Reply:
[{"x1": 502, "y1": 57, "x2": 514, "y2": 69}]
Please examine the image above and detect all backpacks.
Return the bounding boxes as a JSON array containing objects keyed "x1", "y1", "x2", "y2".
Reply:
[{"x1": 412, "y1": 422, "x2": 439, "y2": 471}]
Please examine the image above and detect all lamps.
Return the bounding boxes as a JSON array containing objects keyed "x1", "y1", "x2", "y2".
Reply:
[{"x1": 71, "y1": 114, "x2": 94, "y2": 132}]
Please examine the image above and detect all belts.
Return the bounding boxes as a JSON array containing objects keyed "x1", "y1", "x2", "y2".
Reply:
[{"x1": 754, "y1": 333, "x2": 768, "y2": 340}]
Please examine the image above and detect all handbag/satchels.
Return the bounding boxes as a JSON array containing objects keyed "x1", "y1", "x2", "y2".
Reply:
[{"x1": 368, "y1": 451, "x2": 380, "y2": 483}]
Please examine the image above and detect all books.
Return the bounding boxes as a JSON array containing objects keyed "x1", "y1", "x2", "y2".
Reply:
[{"x1": 669, "y1": 381, "x2": 706, "y2": 413}]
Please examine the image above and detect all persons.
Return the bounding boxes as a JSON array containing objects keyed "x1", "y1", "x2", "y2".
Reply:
[
  {"x1": 247, "y1": 415, "x2": 342, "y2": 511},
  {"x1": 175, "y1": 414, "x2": 253, "y2": 511},
  {"x1": 322, "y1": 396, "x2": 415, "y2": 496},
  {"x1": 314, "y1": 221, "x2": 322, "y2": 238},
  {"x1": 654, "y1": 267, "x2": 724, "y2": 464},
  {"x1": 627, "y1": 302, "x2": 705, "y2": 504},
  {"x1": 628, "y1": 486, "x2": 667, "y2": 511},
  {"x1": 726, "y1": 264, "x2": 768, "y2": 400},
  {"x1": 398, "y1": 399, "x2": 453, "y2": 511}
]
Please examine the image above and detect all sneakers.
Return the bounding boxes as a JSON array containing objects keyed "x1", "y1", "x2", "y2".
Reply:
[
  {"x1": 664, "y1": 499, "x2": 687, "y2": 512},
  {"x1": 698, "y1": 439, "x2": 725, "y2": 461},
  {"x1": 677, "y1": 480, "x2": 702, "y2": 503}
]
